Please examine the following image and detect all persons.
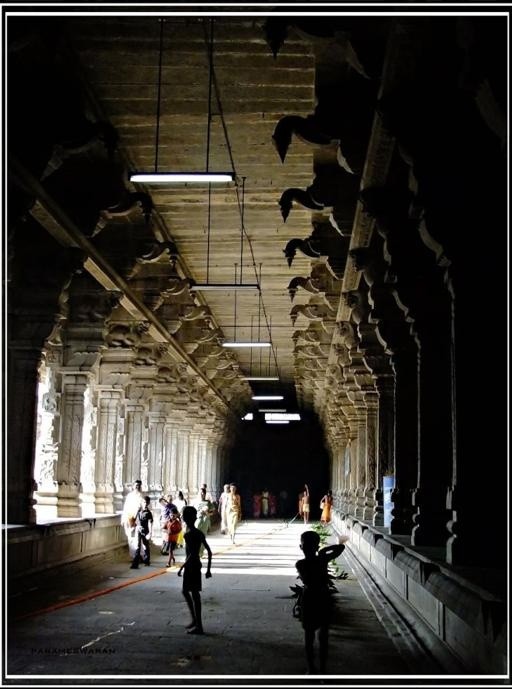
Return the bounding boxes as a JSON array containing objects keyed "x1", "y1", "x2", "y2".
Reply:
[
  {"x1": 295, "y1": 531, "x2": 345, "y2": 663},
  {"x1": 298, "y1": 484, "x2": 333, "y2": 569},
  {"x1": 177, "y1": 506, "x2": 212, "y2": 635},
  {"x1": 120, "y1": 479, "x2": 278, "y2": 569}
]
